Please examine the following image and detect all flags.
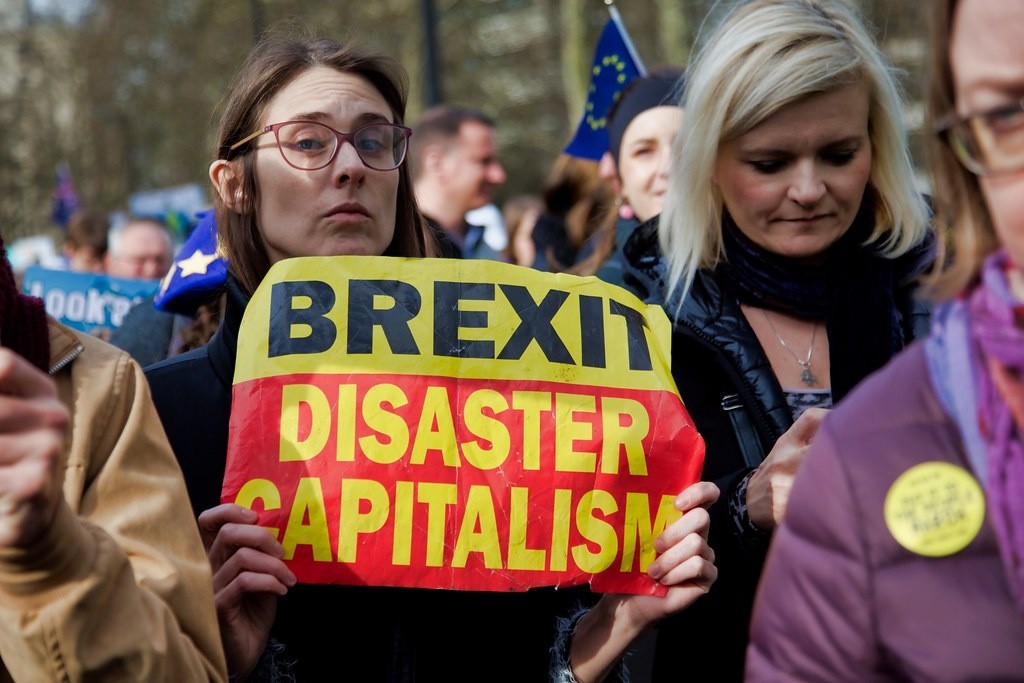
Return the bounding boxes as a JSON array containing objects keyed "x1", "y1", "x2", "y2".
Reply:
[{"x1": 561, "y1": 15, "x2": 642, "y2": 161}]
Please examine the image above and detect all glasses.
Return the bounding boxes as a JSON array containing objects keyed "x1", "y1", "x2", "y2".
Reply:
[
  {"x1": 231, "y1": 121, "x2": 413, "y2": 171},
  {"x1": 933, "y1": 103, "x2": 1024, "y2": 175}
]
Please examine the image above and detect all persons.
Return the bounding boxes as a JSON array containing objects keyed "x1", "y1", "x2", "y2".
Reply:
[
  {"x1": 0, "y1": 1, "x2": 1024, "y2": 683},
  {"x1": 137, "y1": 42, "x2": 719, "y2": 682},
  {"x1": 744, "y1": 1, "x2": 1024, "y2": 683}
]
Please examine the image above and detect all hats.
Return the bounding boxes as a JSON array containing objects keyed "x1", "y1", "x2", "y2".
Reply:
[
  {"x1": 609, "y1": 69, "x2": 688, "y2": 184},
  {"x1": 155, "y1": 208, "x2": 228, "y2": 315}
]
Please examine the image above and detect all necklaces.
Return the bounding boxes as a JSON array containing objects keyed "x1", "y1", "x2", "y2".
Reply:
[{"x1": 763, "y1": 309, "x2": 820, "y2": 387}]
